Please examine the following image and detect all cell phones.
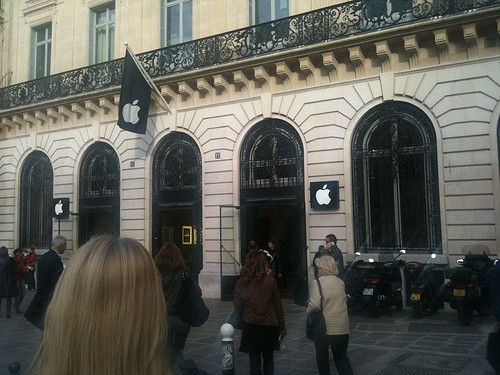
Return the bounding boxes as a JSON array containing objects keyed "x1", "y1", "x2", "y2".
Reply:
[{"x1": 327, "y1": 242, "x2": 335, "y2": 246}]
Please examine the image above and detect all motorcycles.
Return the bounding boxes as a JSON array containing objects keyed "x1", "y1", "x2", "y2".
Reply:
[
  {"x1": 406, "y1": 254, "x2": 457, "y2": 318},
  {"x1": 362, "y1": 249, "x2": 416, "y2": 319},
  {"x1": 437, "y1": 244, "x2": 500, "y2": 323},
  {"x1": 342, "y1": 252, "x2": 376, "y2": 310}
]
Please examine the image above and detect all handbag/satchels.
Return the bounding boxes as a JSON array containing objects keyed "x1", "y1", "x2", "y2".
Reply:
[
  {"x1": 24, "y1": 300, "x2": 49, "y2": 331},
  {"x1": 306, "y1": 311, "x2": 326, "y2": 341},
  {"x1": 227, "y1": 298, "x2": 264, "y2": 329},
  {"x1": 23, "y1": 268, "x2": 34, "y2": 284},
  {"x1": 176, "y1": 269, "x2": 210, "y2": 327}
]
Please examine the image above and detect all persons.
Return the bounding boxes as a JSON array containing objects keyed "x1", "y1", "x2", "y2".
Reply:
[
  {"x1": 0, "y1": 246, "x2": 37, "y2": 321},
  {"x1": 313, "y1": 234, "x2": 343, "y2": 279},
  {"x1": 242, "y1": 240, "x2": 282, "y2": 284},
  {"x1": 228, "y1": 251, "x2": 287, "y2": 375},
  {"x1": 25, "y1": 236, "x2": 66, "y2": 329},
  {"x1": 25, "y1": 231, "x2": 171, "y2": 375},
  {"x1": 305, "y1": 256, "x2": 354, "y2": 375},
  {"x1": 154, "y1": 241, "x2": 210, "y2": 375}
]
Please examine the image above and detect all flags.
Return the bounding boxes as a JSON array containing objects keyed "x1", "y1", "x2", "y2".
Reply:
[{"x1": 116, "y1": 46, "x2": 153, "y2": 135}]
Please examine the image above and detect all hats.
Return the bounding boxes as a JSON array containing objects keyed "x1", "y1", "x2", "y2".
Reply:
[{"x1": 13, "y1": 248, "x2": 23, "y2": 254}]
